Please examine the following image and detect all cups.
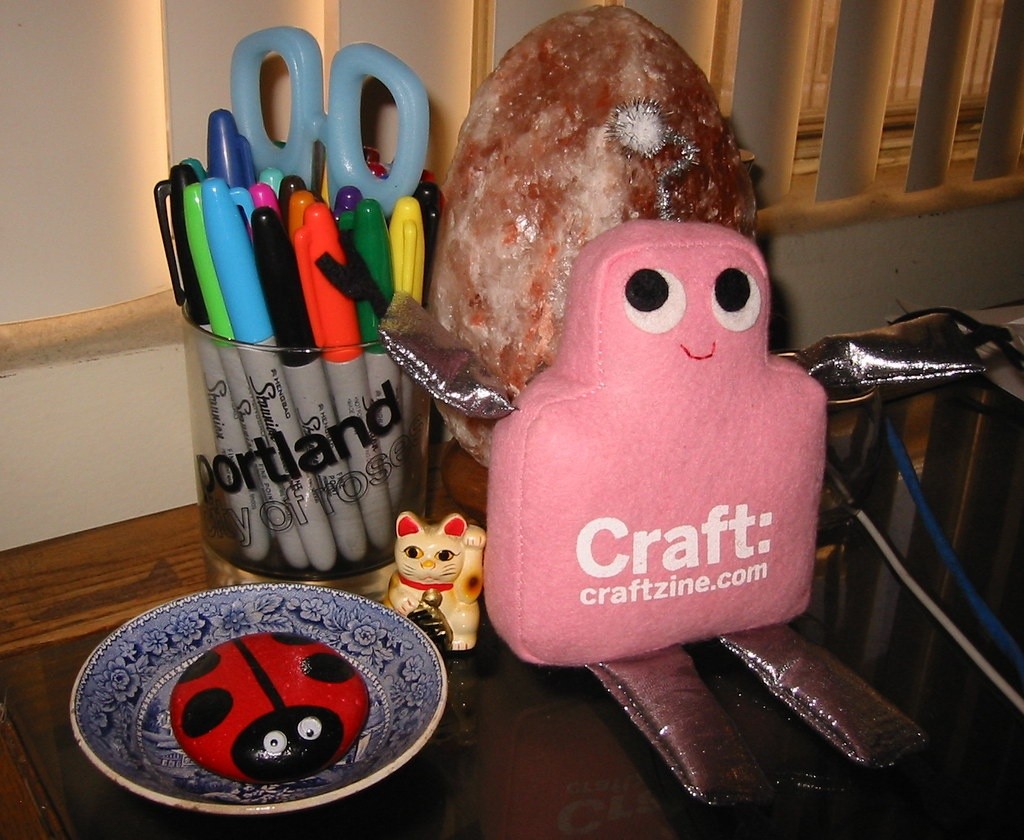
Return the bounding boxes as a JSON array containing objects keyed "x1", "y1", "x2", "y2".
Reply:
[{"x1": 180, "y1": 297, "x2": 432, "y2": 603}]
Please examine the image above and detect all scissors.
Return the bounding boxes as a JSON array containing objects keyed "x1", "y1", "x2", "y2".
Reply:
[{"x1": 231, "y1": 25, "x2": 431, "y2": 220}]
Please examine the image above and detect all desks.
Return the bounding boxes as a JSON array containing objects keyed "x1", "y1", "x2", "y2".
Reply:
[{"x1": 0, "y1": 437, "x2": 863, "y2": 840}]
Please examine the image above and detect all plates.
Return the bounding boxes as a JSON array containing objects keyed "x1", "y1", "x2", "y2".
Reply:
[{"x1": 69, "y1": 581, "x2": 448, "y2": 814}]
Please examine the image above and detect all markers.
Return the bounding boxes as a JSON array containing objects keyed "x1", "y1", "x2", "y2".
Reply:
[{"x1": 153, "y1": 105, "x2": 440, "y2": 580}]
